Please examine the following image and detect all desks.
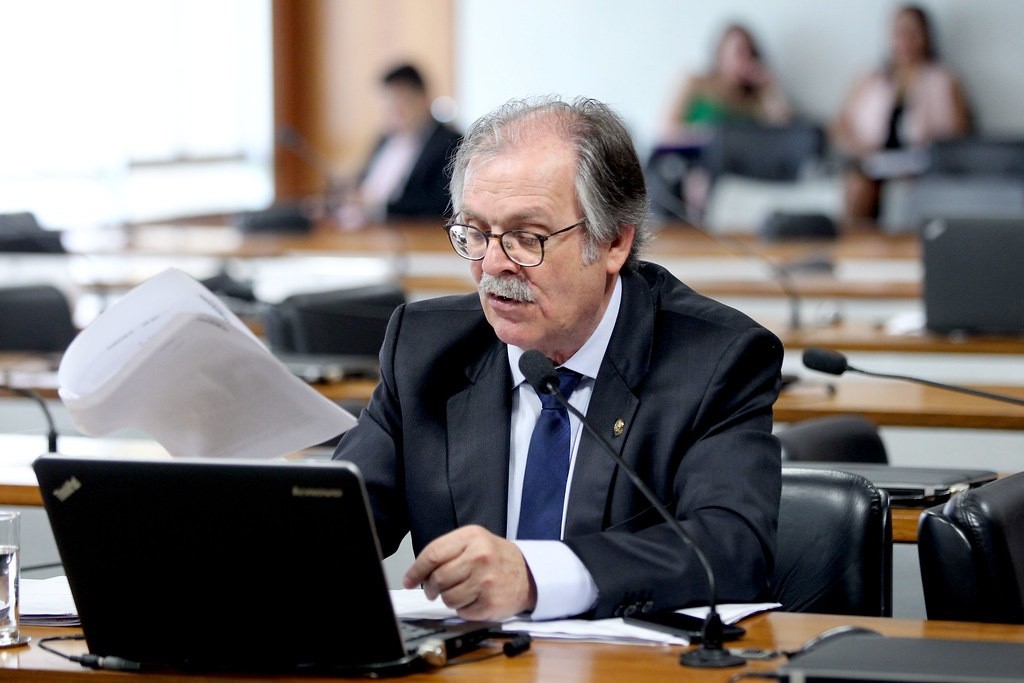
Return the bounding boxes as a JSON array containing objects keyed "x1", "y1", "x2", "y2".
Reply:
[{"x1": 0, "y1": 208, "x2": 1024, "y2": 681}]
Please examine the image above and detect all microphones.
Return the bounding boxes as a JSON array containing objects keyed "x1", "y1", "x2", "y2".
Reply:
[
  {"x1": 801, "y1": 343, "x2": 1023, "y2": 407},
  {"x1": 519, "y1": 347, "x2": 745, "y2": 672}
]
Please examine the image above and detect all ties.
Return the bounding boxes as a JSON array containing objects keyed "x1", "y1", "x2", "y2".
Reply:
[{"x1": 517, "y1": 368, "x2": 584, "y2": 541}]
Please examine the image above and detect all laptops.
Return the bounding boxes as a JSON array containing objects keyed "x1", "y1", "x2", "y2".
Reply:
[
  {"x1": 781, "y1": 460, "x2": 998, "y2": 511},
  {"x1": 28, "y1": 454, "x2": 503, "y2": 681},
  {"x1": 773, "y1": 634, "x2": 1023, "y2": 683}
]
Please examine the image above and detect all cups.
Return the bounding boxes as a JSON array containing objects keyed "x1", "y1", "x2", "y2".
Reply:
[{"x1": 0, "y1": 510, "x2": 23, "y2": 647}]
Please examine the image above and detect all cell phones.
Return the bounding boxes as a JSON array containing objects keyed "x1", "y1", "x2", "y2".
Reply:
[{"x1": 622, "y1": 609, "x2": 745, "y2": 647}]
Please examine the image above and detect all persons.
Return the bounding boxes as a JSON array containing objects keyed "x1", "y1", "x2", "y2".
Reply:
[
  {"x1": 328, "y1": 95, "x2": 783, "y2": 633},
  {"x1": 670, "y1": 23, "x2": 791, "y2": 169},
  {"x1": 833, "y1": 6, "x2": 974, "y2": 221},
  {"x1": 337, "y1": 65, "x2": 467, "y2": 223}
]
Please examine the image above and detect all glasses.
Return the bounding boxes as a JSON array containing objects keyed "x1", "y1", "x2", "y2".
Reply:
[{"x1": 443, "y1": 210, "x2": 587, "y2": 267}]
